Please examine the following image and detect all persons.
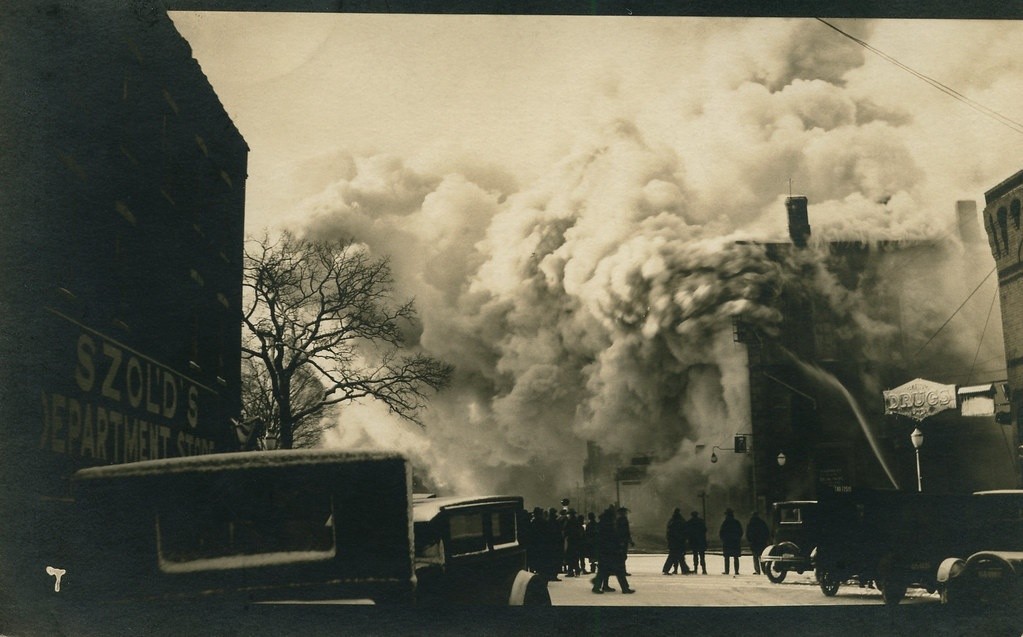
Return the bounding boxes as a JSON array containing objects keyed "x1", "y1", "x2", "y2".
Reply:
[
  {"x1": 719, "y1": 507, "x2": 743, "y2": 575},
  {"x1": 662, "y1": 507, "x2": 709, "y2": 575},
  {"x1": 520, "y1": 504, "x2": 637, "y2": 595},
  {"x1": 746, "y1": 510, "x2": 771, "y2": 574}
]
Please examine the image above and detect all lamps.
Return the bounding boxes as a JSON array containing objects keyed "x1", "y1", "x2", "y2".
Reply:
[
  {"x1": 776, "y1": 449, "x2": 790, "y2": 467},
  {"x1": 710, "y1": 446, "x2": 749, "y2": 463},
  {"x1": 909, "y1": 426, "x2": 925, "y2": 451}
]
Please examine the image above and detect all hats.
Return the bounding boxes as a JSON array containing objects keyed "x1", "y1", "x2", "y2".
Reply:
[{"x1": 724, "y1": 508, "x2": 735, "y2": 514}]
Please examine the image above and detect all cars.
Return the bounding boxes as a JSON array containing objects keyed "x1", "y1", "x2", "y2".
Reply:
[
  {"x1": 71, "y1": 450, "x2": 420, "y2": 618},
  {"x1": 761, "y1": 500, "x2": 822, "y2": 585},
  {"x1": 405, "y1": 493, "x2": 551, "y2": 614}
]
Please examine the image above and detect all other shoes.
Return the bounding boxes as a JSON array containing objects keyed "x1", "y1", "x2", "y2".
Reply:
[
  {"x1": 622, "y1": 588, "x2": 635, "y2": 594},
  {"x1": 602, "y1": 587, "x2": 615, "y2": 592},
  {"x1": 592, "y1": 589, "x2": 604, "y2": 594}
]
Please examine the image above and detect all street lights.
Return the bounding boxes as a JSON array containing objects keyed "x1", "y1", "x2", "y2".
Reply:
[{"x1": 910, "y1": 427, "x2": 925, "y2": 493}]
